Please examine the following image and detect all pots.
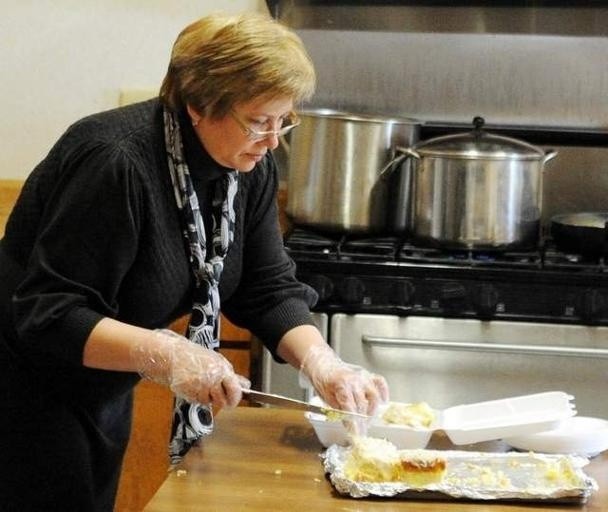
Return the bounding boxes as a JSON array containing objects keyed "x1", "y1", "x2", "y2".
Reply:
[
  {"x1": 279, "y1": 106, "x2": 418, "y2": 241},
  {"x1": 550, "y1": 207, "x2": 607, "y2": 255},
  {"x1": 395, "y1": 117, "x2": 559, "y2": 251}
]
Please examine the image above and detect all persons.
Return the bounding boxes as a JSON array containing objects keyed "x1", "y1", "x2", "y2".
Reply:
[{"x1": 0, "y1": 13, "x2": 390, "y2": 512}]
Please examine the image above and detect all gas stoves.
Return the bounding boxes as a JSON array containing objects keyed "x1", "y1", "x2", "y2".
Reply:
[{"x1": 283, "y1": 221, "x2": 606, "y2": 326}]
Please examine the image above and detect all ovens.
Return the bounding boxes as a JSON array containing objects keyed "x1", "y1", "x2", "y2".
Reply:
[{"x1": 259, "y1": 306, "x2": 608, "y2": 423}]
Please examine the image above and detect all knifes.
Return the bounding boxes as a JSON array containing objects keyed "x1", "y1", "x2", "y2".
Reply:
[{"x1": 238, "y1": 386, "x2": 373, "y2": 424}]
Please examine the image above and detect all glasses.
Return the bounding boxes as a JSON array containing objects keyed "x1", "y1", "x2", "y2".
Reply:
[{"x1": 226, "y1": 108, "x2": 301, "y2": 144}]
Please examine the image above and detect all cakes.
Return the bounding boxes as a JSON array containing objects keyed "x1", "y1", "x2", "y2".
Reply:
[
  {"x1": 384, "y1": 402, "x2": 432, "y2": 430},
  {"x1": 344, "y1": 439, "x2": 447, "y2": 483}
]
